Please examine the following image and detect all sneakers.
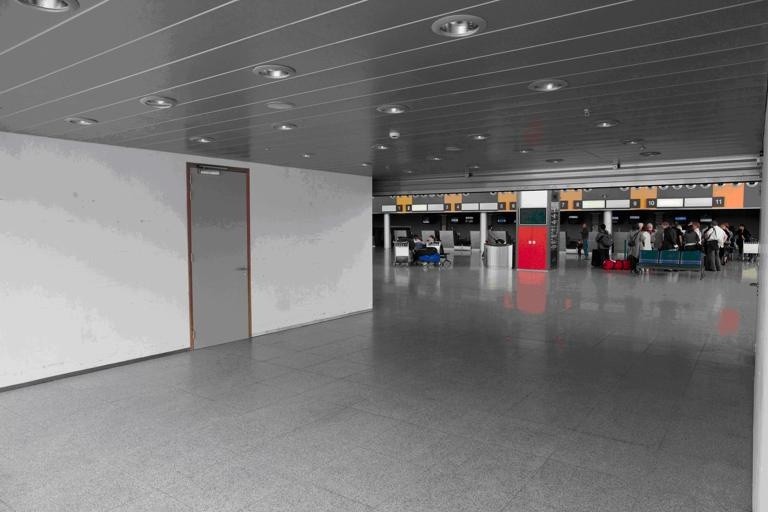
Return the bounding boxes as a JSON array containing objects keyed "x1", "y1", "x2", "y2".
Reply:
[{"x1": 704, "y1": 258, "x2": 730, "y2": 273}]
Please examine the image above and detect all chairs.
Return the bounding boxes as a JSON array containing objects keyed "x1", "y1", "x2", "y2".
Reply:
[{"x1": 635, "y1": 250, "x2": 705, "y2": 280}]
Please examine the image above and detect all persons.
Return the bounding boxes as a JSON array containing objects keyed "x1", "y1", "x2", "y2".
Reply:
[
  {"x1": 577, "y1": 239, "x2": 583, "y2": 256},
  {"x1": 595, "y1": 224, "x2": 613, "y2": 266},
  {"x1": 482, "y1": 225, "x2": 498, "y2": 257},
  {"x1": 735, "y1": 224, "x2": 753, "y2": 259},
  {"x1": 580, "y1": 224, "x2": 590, "y2": 259},
  {"x1": 427, "y1": 234, "x2": 435, "y2": 241},
  {"x1": 414, "y1": 235, "x2": 422, "y2": 242},
  {"x1": 629, "y1": 218, "x2": 737, "y2": 276}
]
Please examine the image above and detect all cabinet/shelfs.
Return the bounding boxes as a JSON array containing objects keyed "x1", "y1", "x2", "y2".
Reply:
[{"x1": 517, "y1": 225, "x2": 547, "y2": 272}]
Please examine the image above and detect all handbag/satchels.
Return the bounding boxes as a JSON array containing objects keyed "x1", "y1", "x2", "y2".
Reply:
[
  {"x1": 703, "y1": 240, "x2": 719, "y2": 252},
  {"x1": 627, "y1": 238, "x2": 636, "y2": 247}
]
